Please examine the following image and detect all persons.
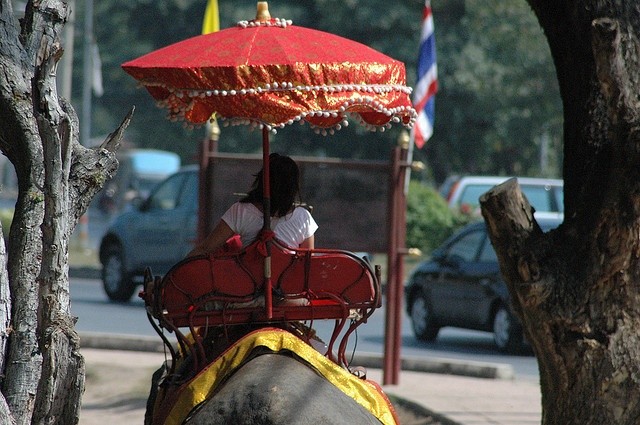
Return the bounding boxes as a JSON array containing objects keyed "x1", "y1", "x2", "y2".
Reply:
[{"x1": 172, "y1": 152, "x2": 318, "y2": 258}]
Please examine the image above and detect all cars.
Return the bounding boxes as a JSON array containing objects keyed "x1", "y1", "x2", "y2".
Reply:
[{"x1": 403, "y1": 209, "x2": 563, "y2": 352}]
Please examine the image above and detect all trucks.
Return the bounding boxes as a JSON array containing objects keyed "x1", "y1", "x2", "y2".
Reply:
[{"x1": 97, "y1": 147, "x2": 183, "y2": 215}]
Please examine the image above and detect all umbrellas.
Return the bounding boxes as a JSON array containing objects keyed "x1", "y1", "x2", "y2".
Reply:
[{"x1": 119, "y1": 1, "x2": 418, "y2": 231}]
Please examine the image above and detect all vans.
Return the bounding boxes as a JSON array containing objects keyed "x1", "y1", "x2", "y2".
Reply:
[{"x1": 433, "y1": 173, "x2": 566, "y2": 234}]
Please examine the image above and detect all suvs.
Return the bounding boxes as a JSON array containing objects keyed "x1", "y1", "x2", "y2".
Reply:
[{"x1": 98, "y1": 165, "x2": 202, "y2": 305}]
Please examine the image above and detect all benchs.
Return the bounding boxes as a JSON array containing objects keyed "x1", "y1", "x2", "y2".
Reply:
[{"x1": 138, "y1": 228, "x2": 384, "y2": 386}]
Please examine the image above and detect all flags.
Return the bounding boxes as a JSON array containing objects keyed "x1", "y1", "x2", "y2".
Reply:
[{"x1": 412, "y1": 2, "x2": 439, "y2": 148}]
